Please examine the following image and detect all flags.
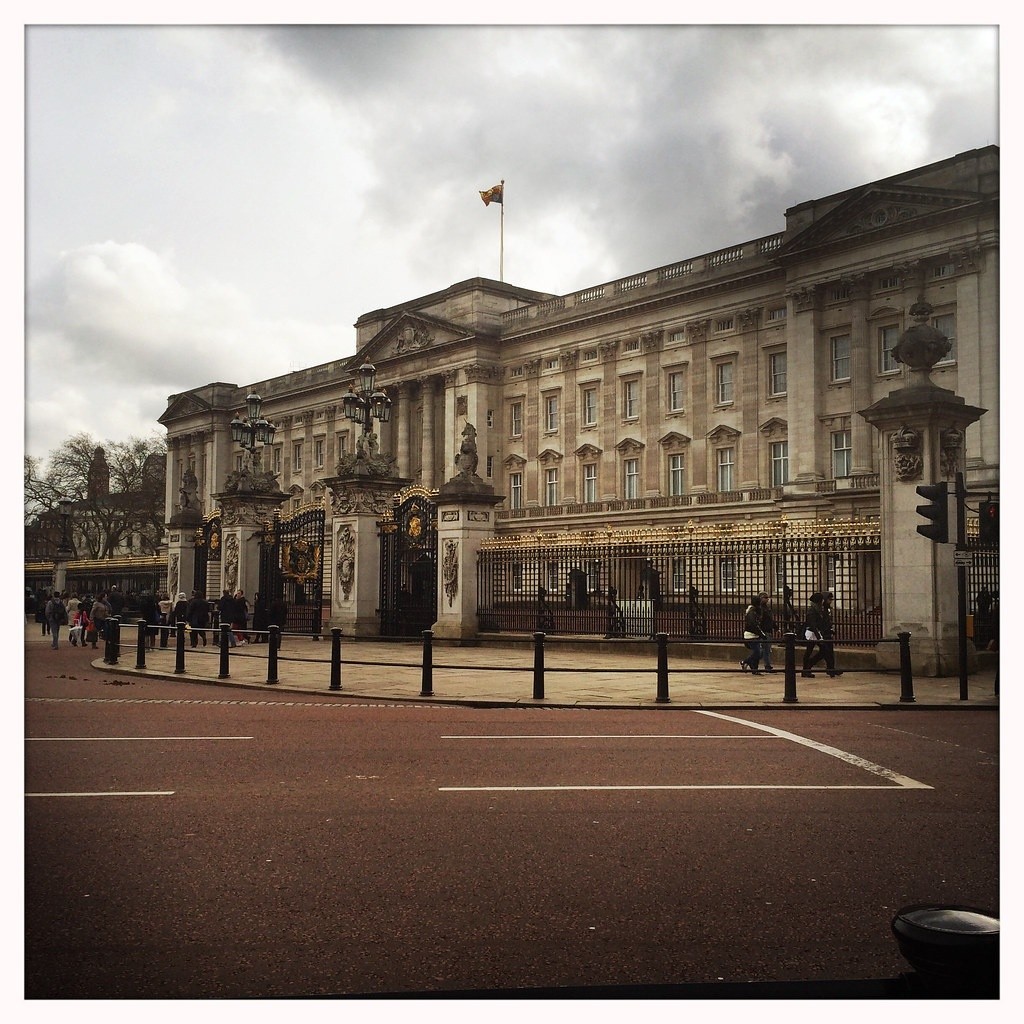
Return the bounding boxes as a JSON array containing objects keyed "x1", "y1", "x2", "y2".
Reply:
[{"x1": 479, "y1": 180, "x2": 503, "y2": 206}]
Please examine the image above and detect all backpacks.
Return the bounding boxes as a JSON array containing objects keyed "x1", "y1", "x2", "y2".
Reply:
[{"x1": 51, "y1": 598, "x2": 67, "y2": 626}]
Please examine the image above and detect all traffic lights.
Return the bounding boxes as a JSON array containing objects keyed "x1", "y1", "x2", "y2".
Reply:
[
  {"x1": 915, "y1": 482, "x2": 949, "y2": 544},
  {"x1": 978, "y1": 500, "x2": 1000, "y2": 543}
]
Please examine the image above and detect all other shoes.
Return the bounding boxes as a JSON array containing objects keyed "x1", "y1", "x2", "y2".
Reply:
[
  {"x1": 751, "y1": 669, "x2": 765, "y2": 675},
  {"x1": 765, "y1": 665, "x2": 773, "y2": 669},
  {"x1": 826, "y1": 668, "x2": 843, "y2": 677},
  {"x1": 740, "y1": 660, "x2": 748, "y2": 673},
  {"x1": 203, "y1": 638, "x2": 207, "y2": 646},
  {"x1": 252, "y1": 639, "x2": 259, "y2": 644},
  {"x1": 801, "y1": 658, "x2": 815, "y2": 678},
  {"x1": 51, "y1": 644, "x2": 58, "y2": 650},
  {"x1": 248, "y1": 636, "x2": 251, "y2": 644}
]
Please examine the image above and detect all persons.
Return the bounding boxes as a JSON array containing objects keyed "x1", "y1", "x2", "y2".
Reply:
[
  {"x1": 31, "y1": 585, "x2": 126, "y2": 651},
  {"x1": 801, "y1": 592, "x2": 843, "y2": 677},
  {"x1": 137, "y1": 589, "x2": 287, "y2": 652},
  {"x1": 738, "y1": 592, "x2": 782, "y2": 675},
  {"x1": 987, "y1": 583, "x2": 999, "y2": 698}
]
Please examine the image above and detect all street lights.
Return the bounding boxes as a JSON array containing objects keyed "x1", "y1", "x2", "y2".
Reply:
[
  {"x1": 230, "y1": 387, "x2": 278, "y2": 454},
  {"x1": 55, "y1": 496, "x2": 78, "y2": 558},
  {"x1": 341, "y1": 355, "x2": 394, "y2": 437}
]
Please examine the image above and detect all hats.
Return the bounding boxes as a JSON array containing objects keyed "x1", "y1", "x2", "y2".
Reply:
[
  {"x1": 810, "y1": 593, "x2": 823, "y2": 603},
  {"x1": 161, "y1": 594, "x2": 169, "y2": 600}
]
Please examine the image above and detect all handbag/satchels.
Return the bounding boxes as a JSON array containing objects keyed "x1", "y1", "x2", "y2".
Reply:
[
  {"x1": 86, "y1": 620, "x2": 98, "y2": 642},
  {"x1": 168, "y1": 603, "x2": 173, "y2": 624},
  {"x1": 183, "y1": 622, "x2": 192, "y2": 633},
  {"x1": 796, "y1": 622, "x2": 806, "y2": 640}
]
